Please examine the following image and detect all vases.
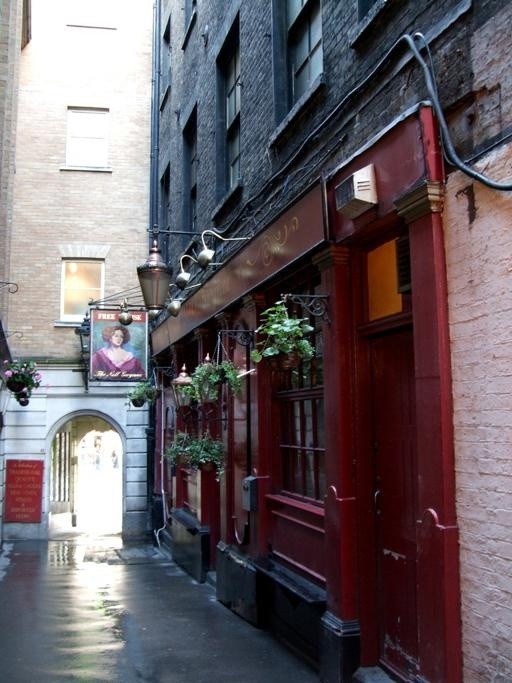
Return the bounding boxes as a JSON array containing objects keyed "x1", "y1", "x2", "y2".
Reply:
[{"x1": 19, "y1": 394, "x2": 29, "y2": 409}]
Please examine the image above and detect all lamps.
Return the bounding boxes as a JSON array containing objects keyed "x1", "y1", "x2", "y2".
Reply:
[
  {"x1": 173, "y1": 367, "x2": 193, "y2": 410},
  {"x1": 116, "y1": 223, "x2": 253, "y2": 328}
]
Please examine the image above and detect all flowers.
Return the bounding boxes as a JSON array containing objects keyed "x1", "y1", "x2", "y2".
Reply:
[{"x1": 2, "y1": 358, "x2": 44, "y2": 390}]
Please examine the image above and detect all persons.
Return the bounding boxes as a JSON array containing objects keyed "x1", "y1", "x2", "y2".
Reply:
[{"x1": 92, "y1": 324, "x2": 144, "y2": 380}]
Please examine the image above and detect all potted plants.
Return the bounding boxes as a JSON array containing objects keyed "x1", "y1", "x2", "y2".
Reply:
[
  {"x1": 193, "y1": 440, "x2": 227, "y2": 478},
  {"x1": 163, "y1": 431, "x2": 199, "y2": 469},
  {"x1": 258, "y1": 306, "x2": 315, "y2": 369},
  {"x1": 193, "y1": 353, "x2": 246, "y2": 403}
]
